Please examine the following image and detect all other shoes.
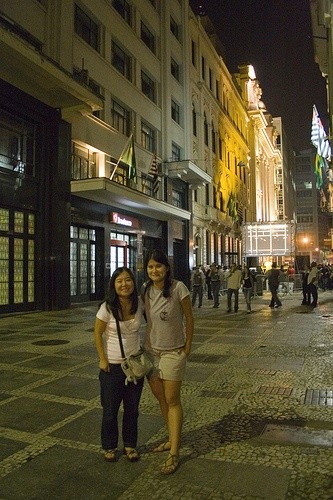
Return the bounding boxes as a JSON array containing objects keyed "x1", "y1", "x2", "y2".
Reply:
[
  {"x1": 105, "y1": 449, "x2": 116, "y2": 461},
  {"x1": 247, "y1": 309, "x2": 251, "y2": 313},
  {"x1": 217, "y1": 303, "x2": 220, "y2": 308},
  {"x1": 301, "y1": 303, "x2": 306, "y2": 305},
  {"x1": 198, "y1": 305, "x2": 200, "y2": 308},
  {"x1": 125, "y1": 446, "x2": 138, "y2": 461},
  {"x1": 227, "y1": 309, "x2": 232, "y2": 312},
  {"x1": 234, "y1": 308, "x2": 238, "y2": 312},
  {"x1": 307, "y1": 301, "x2": 311, "y2": 305},
  {"x1": 213, "y1": 306, "x2": 215, "y2": 308},
  {"x1": 275, "y1": 304, "x2": 282, "y2": 308},
  {"x1": 269, "y1": 304, "x2": 274, "y2": 307},
  {"x1": 311, "y1": 303, "x2": 317, "y2": 306}
]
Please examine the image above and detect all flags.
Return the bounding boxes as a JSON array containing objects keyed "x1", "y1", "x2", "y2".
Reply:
[
  {"x1": 147, "y1": 153, "x2": 163, "y2": 195},
  {"x1": 311, "y1": 108, "x2": 332, "y2": 162},
  {"x1": 228, "y1": 194, "x2": 239, "y2": 224},
  {"x1": 121, "y1": 134, "x2": 137, "y2": 184},
  {"x1": 315, "y1": 154, "x2": 324, "y2": 190}
]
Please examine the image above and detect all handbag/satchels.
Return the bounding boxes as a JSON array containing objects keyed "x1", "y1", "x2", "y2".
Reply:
[{"x1": 122, "y1": 349, "x2": 153, "y2": 385}]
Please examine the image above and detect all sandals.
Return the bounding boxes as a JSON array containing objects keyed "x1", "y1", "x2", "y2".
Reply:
[
  {"x1": 161, "y1": 453, "x2": 179, "y2": 474},
  {"x1": 154, "y1": 440, "x2": 173, "y2": 452}
]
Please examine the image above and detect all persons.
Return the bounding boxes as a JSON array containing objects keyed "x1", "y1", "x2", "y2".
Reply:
[
  {"x1": 140, "y1": 250, "x2": 194, "y2": 474},
  {"x1": 241, "y1": 265, "x2": 255, "y2": 313},
  {"x1": 307, "y1": 261, "x2": 319, "y2": 309},
  {"x1": 191, "y1": 266, "x2": 206, "y2": 308},
  {"x1": 280, "y1": 262, "x2": 333, "y2": 292},
  {"x1": 224, "y1": 262, "x2": 242, "y2": 314},
  {"x1": 207, "y1": 263, "x2": 221, "y2": 308},
  {"x1": 262, "y1": 261, "x2": 283, "y2": 308},
  {"x1": 190, "y1": 264, "x2": 256, "y2": 300},
  {"x1": 300, "y1": 263, "x2": 312, "y2": 306},
  {"x1": 95, "y1": 267, "x2": 153, "y2": 463}
]
